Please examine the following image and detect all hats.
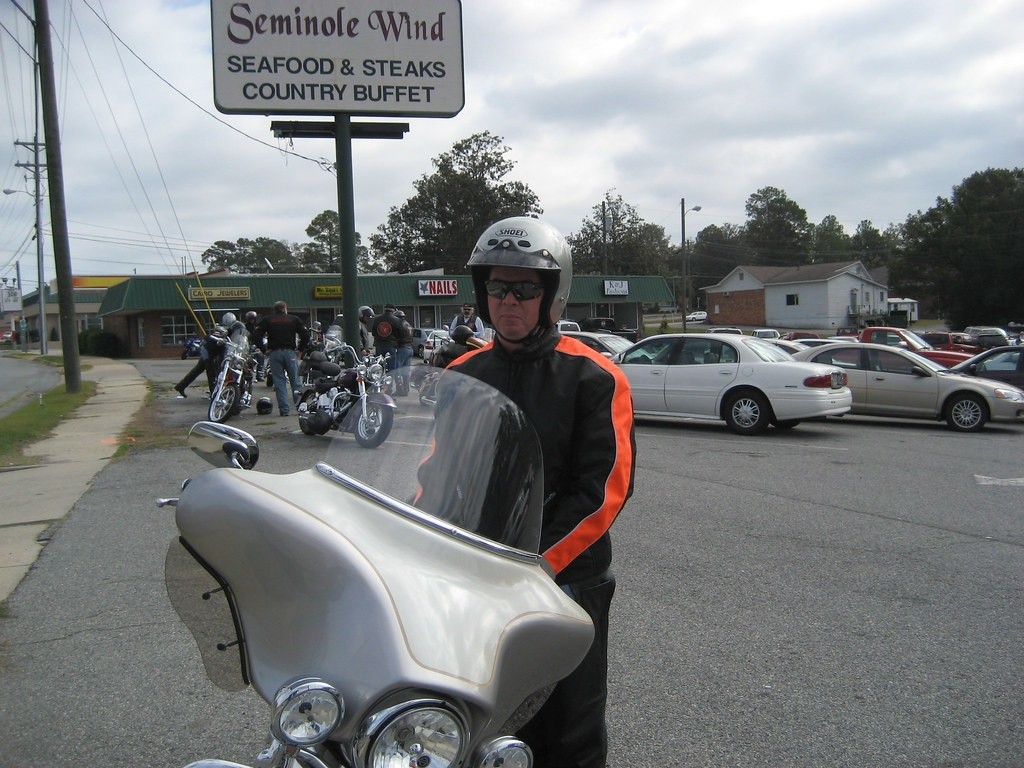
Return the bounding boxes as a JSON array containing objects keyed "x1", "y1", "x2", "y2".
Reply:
[
  {"x1": 463, "y1": 303, "x2": 472, "y2": 307},
  {"x1": 384, "y1": 304, "x2": 394, "y2": 309}
]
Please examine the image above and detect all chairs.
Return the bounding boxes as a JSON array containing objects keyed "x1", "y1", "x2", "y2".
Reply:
[
  {"x1": 679, "y1": 351, "x2": 701, "y2": 365},
  {"x1": 870, "y1": 350, "x2": 882, "y2": 371},
  {"x1": 704, "y1": 351, "x2": 719, "y2": 363}
]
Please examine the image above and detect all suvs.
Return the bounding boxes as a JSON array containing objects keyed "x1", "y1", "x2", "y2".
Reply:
[
  {"x1": 704, "y1": 327, "x2": 742, "y2": 335},
  {"x1": 963, "y1": 326, "x2": 1010, "y2": 348}
]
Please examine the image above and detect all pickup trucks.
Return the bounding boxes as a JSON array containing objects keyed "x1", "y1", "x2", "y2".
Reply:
[
  {"x1": 828, "y1": 327, "x2": 982, "y2": 375},
  {"x1": 555, "y1": 317, "x2": 638, "y2": 347}
]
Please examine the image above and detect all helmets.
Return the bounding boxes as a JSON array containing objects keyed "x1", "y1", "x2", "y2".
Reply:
[
  {"x1": 451, "y1": 325, "x2": 475, "y2": 346},
  {"x1": 394, "y1": 310, "x2": 405, "y2": 317},
  {"x1": 222, "y1": 313, "x2": 236, "y2": 326},
  {"x1": 257, "y1": 396, "x2": 273, "y2": 414},
  {"x1": 467, "y1": 217, "x2": 573, "y2": 329},
  {"x1": 359, "y1": 306, "x2": 374, "y2": 318},
  {"x1": 246, "y1": 311, "x2": 257, "y2": 319}
]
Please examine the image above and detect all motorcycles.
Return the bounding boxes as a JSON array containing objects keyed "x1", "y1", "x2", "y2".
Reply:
[
  {"x1": 208, "y1": 325, "x2": 290, "y2": 425},
  {"x1": 181, "y1": 337, "x2": 204, "y2": 360},
  {"x1": 158, "y1": 363, "x2": 595, "y2": 768},
  {"x1": 296, "y1": 312, "x2": 441, "y2": 450}
]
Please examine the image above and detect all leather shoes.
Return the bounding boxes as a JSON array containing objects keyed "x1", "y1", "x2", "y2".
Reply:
[{"x1": 173, "y1": 386, "x2": 187, "y2": 398}]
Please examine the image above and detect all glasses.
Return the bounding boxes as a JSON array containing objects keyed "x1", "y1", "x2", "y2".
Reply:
[
  {"x1": 464, "y1": 308, "x2": 474, "y2": 311},
  {"x1": 484, "y1": 279, "x2": 545, "y2": 302}
]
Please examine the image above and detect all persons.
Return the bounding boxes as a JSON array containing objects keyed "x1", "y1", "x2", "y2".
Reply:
[
  {"x1": 411, "y1": 216, "x2": 638, "y2": 768},
  {"x1": 174, "y1": 321, "x2": 245, "y2": 398},
  {"x1": 359, "y1": 303, "x2": 413, "y2": 396},
  {"x1": 449, "y1": 303, "x2": 485, "y2": 338},
  {"x1": 245, "y1": 310, "x2": 266, "y2": 381},
  {"x1": 253, "y1": 300, "x2": 310, "y2": 416}
]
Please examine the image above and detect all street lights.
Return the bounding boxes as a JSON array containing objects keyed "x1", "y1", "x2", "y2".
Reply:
[
  {"x1": 4, "y1": 189, "x2": 47, "y2": 354},
  {"x1": 681, "y1": 205, "x2": 701, "y2": 332}
]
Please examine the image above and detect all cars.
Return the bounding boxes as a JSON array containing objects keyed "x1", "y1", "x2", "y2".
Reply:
[
  {"x1": 3, "y1": 331, "x2": 13, "y2": 338},
  {"x1": 556, "y1": 333, "x2": 853, "y2": 436},
  {"x1": 686, "y1": 311, "x2": 707, "y2": 322},
  {"x1": 949, "y1": 346, "x2": 1024, "y2": 392},
  {"x1": 412, "y1": 328, "x2": 451, "y2": 364},
  {"x1": 789, "y1": 341, "x2": 1024, "y2": 433},
  {"x1": 752, "y1": 329, "x2": 859, "y2": 357}
]
